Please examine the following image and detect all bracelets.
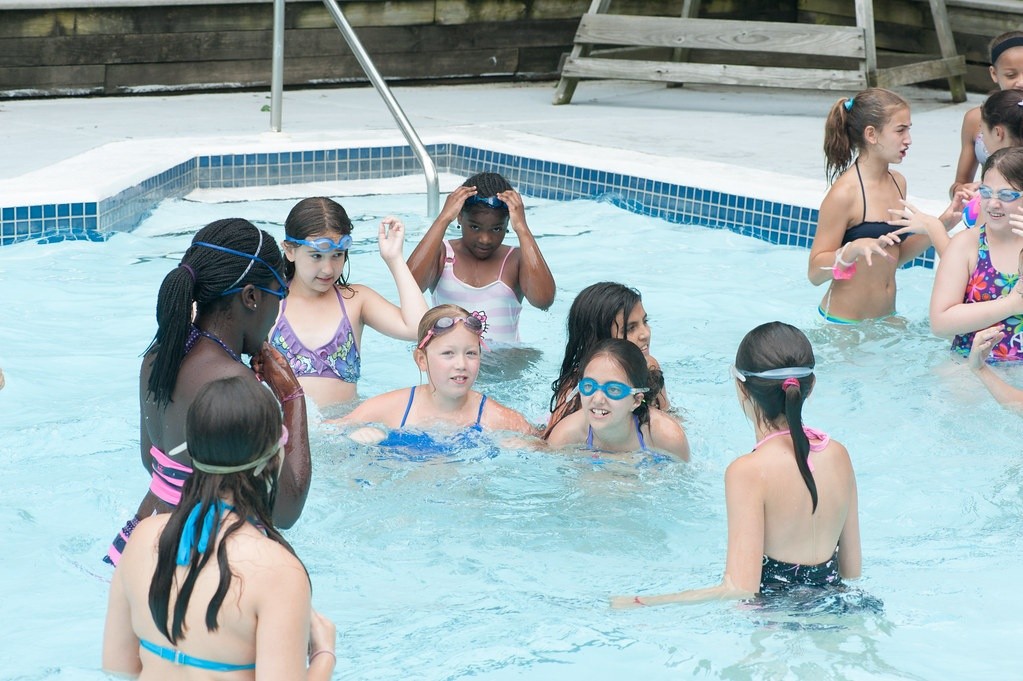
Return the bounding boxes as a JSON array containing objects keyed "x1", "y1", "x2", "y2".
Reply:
[
  {"x1": 282, "y1": 386, "x2": 305, "y2": 402},
  {"x1": 953, "y1": 185, "x2": 958, "y2": 197},
  {"x1": 592, "y1": 451, "x2": 601, "y2": 458},
  {"x1": 820, "y1": 242, "x2": 859, "y2": 281},
  {"x1": 635, "y1": 595, "x2": 644, "y2": 607},
  {"x1": 309, "y1": 649, "x2": 337, "y2": 663}
]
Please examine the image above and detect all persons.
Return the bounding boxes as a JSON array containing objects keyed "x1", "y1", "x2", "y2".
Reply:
[
  {"x1": 808, "y1": 87, "x2": 980, "y2": 326},
  {"x1": 929, "y1": 29, "x2": 1023, "y2": 420},
  {"x1": 548, "y1": 337, "x2": 690, "y2": 459},
  {"x1": 544, "y1": 281, "x2": 671, "y2": 438},
  {"x1": 612, "y1": 321, "x2": 862, "y2": 608},
  {"x1": 102, "y1": 374, "x2": 336, "y2": 681},
  {"x1": 267, "y1": 196, "x2": 430, "y2": 407},
  {"x1": 102, "y1": 218, "x2": 312, "y2": 569},
  {"x1": 323, "y1": 303, "x2": 540, "y2": 448},
  {"x1": 406, "y1": 172, "x2": 556, "y2": 342}
]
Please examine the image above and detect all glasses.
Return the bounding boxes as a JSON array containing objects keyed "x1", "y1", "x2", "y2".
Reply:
[
  {"x1": 577, "y1": 377, "x2": 651, "y2": 401},
  {"x1": 978, "y1": 184, "x2": 1023, "y2": 202},
  {"x1": 286, "y1": 236, "x2": 354, "y2": 254},
  {"x1": 418, "y1": 311, "x2": 489, "y2": 349},
  {"x1": 466, "y1": 194, "x2": 504, "y2": 209}
]
